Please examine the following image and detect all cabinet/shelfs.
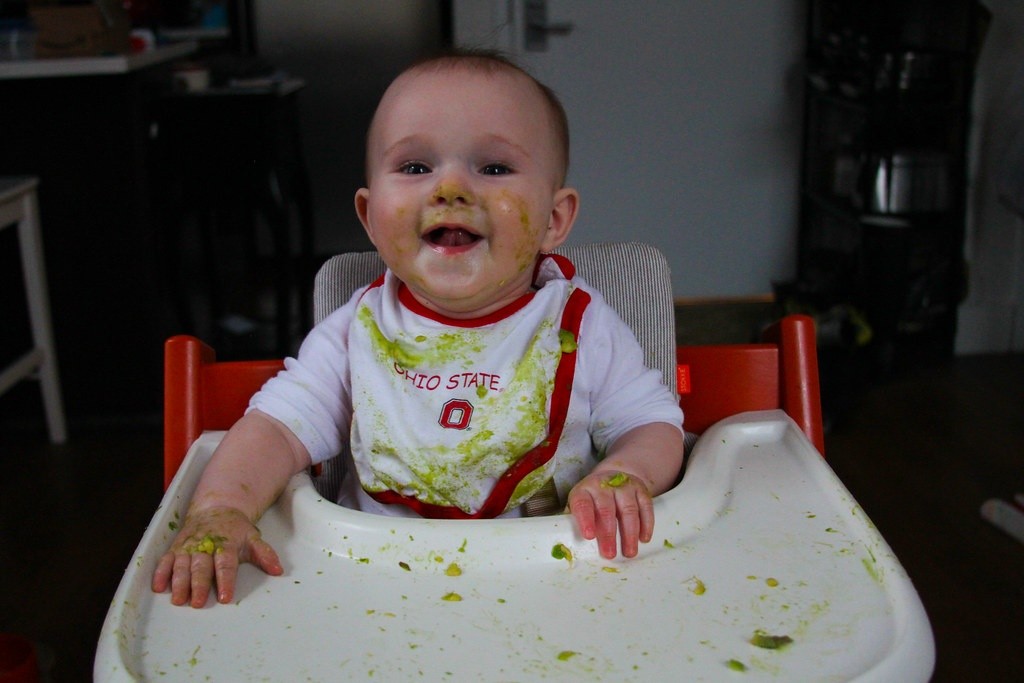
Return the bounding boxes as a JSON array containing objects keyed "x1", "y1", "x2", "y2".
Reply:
[{"x1": 793, "y1": 2, "x2": 993, "y2": 376}]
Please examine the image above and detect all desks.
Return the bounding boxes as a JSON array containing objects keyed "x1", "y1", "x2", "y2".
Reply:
[{"x1": 0, "y1": 46, "x2": 311, "y2": 409}]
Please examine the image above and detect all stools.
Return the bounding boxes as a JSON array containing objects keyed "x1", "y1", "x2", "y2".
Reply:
[{"x1": 0, "y1": 172, "x2": 67, "y2": 444}]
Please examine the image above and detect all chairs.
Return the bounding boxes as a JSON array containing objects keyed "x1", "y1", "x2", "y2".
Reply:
[{"x1": 92, "y1": 246, "x2": 938, "y2": 683}]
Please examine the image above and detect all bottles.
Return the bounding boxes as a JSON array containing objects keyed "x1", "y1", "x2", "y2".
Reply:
[{"x1": 875, "y1": 155, "x2": 916, "y2": 215}]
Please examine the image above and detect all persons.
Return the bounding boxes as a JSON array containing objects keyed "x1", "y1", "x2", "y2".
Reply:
[{"x1": 151, "y1": 49, "x2": 686, "y2": 608}]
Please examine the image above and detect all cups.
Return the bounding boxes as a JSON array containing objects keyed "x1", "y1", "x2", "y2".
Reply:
[
  {"x1": 127, "y1": 29, "x2": 156, "y2": 56},
  {"x1": 172, "y1": 64, "x2": 210, "y2": 94}
]
